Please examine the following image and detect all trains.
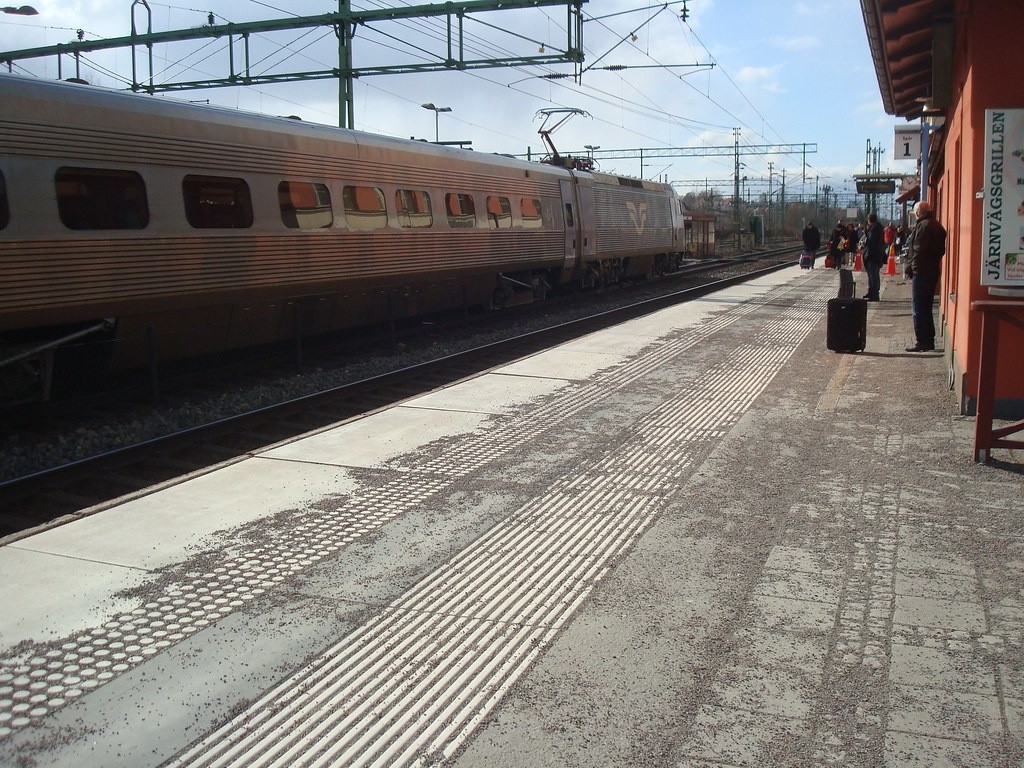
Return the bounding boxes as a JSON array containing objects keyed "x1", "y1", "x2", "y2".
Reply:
[{"x1": 0, "y1": 73, "x2": 685, "y2": 414}]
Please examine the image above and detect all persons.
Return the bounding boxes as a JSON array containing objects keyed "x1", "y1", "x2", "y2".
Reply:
[
  {"x1": 904, "y1": 201, "x2": 946, "y2": 351},
  {"x1": 802, "y1": 221, "x2": 821, "y2": 269},
  {"x1": 828, "y1": 219, "x2": 912, "y2": 270},
  {"x1": 862, "y1": 213, "x2": 885, "y2": 301}
]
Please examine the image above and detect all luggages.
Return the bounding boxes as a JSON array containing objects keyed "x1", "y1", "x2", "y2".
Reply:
[
  {"x1": 827, "y1": 281, "x2": 867, "y2": 351},
  {"x1": 799, "y1": 250, "x2": 813, "y2": 269},
  {"x1": 825, "y1": 252, "x2": 835, "y2": 268}
]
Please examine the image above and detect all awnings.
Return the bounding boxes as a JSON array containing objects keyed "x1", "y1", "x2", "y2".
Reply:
[{"x1": 895, "y1": 185, "x2": 920, "y2": 204}]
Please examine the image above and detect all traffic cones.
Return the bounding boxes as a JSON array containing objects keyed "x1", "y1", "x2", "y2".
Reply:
[
  {"x1": 884, "y1": 245, "x2": 900, "y2": 274},
  {"x1": 852, "y1": 250, "x2": 864, "y2": 271}
]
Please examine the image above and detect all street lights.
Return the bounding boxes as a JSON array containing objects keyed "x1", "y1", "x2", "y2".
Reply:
[
  {"x1": 584, "y1": 145, "x2": 600, "y2": 168},
  {"x1": 421, "y1": 103, "x2": 452, "y2": 142}
]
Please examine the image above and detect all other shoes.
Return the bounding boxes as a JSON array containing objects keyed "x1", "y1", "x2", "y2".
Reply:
[
  {"x1": 905, "y1": 342, "x2": 935, "y2": 352},
  {"x1": 867, "y1": 298, "x2": 879, "y2": 301},
  {"x1": 863, "y1": 294, "x2": 869, "y2": 298},
  {"x1": 837, "y1": 266, "x2": 841, "y2": 270}
]
